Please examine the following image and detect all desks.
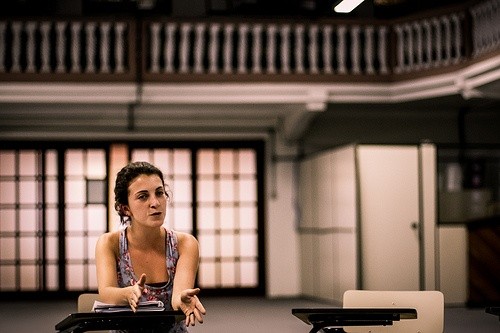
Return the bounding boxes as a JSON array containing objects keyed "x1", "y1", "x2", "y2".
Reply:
[
  {"x1": 290, "y1": 307, "x2": 418, "y2": 333},
  {"x1": 54, "y1": 311, "x2": 187, "y2": 332}
]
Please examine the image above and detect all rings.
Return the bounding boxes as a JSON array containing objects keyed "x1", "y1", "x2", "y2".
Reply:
[{"x1": 189, "y1": 312, "x2": 195, "y2": 315}]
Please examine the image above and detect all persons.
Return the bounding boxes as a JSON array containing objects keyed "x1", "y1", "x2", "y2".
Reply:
[{"x1": 94, "y1": 160, "x2": 209, "y2": 333}]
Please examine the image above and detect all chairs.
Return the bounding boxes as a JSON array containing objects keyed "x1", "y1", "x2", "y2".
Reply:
[
  {"x1": 78, "y1": 294, "x2": 115, "y2": 333},
  {"x1": 342, "y1": 290, "x2": 444, "y2": 332}
]
